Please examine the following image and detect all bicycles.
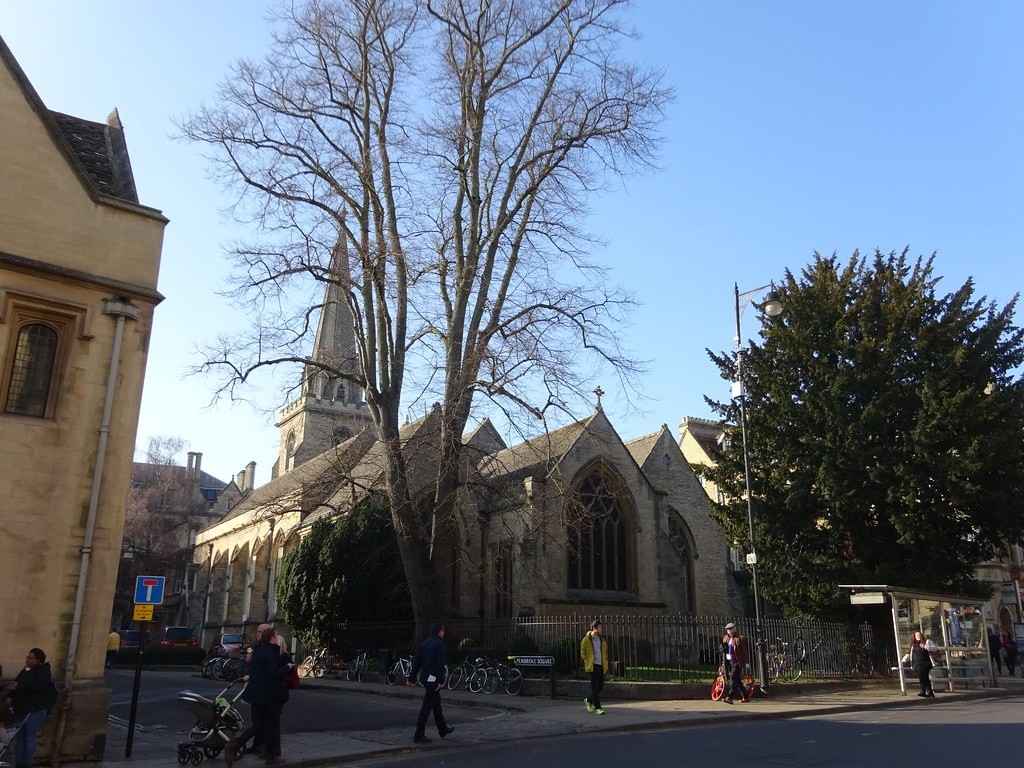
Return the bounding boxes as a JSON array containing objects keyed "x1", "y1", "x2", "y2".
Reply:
[
  {"x1": 818, "y1": 641, "x2": 880, "y2": 678},
  {"x1": 200, "y1": 654, "x2": 247, "y2": 683},
  {"x1": 299, "y1": 643, "x2": 332, "y2": 678},
  {"x1": 754, "y1": 635, "x2": 806, "y2": 682},
  {"x1": 387, "y1": 652, "x2": 523, "y2": 697},
  {"x1": 711, "y1": 650, "x2": 755, "y2": 702},
  {"x1": 346, "y1": 649, "x2": 371, "y2": 682}
]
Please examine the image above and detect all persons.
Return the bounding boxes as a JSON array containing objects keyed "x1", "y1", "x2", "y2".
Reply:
[
  {"x1": 909, "y1": 631, "x2": 938, "y2": 698},
  {"x1": 4, "y1": 647, "x2": 52, "y2": 768},
  {"x1": 580, "y1": 620, "x2": 609, "y2": 715},
  {"x1": 106, "y1": 627, "x2": 120, "y2": 670},
  {"x1": 987, "y1": 627, "x2": 1016, "y2": 676},
  {"x1": 721, "y1": 623, "x2": 751, "y2": 704},
  {"x1": 407, "y1": 624, "x2": 456, "y2": 743},
  {"x1": 242, "y1": 623, "x2": 300, "y2": 765}
]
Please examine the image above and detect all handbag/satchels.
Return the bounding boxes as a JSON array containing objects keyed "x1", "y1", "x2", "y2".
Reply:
[
  {"x1": 923, "y1": 639, "x2": 941, "y2": 663},
  {"x1": 1001, "y1": 647, "x2": 1008, "y2": 659}
]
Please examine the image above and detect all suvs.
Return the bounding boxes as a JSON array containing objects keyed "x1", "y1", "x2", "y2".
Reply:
[
  {"x1": 118, "y1": 630, "x2": 149, "y2": 647},
  {"x1": 208, "y1": 634, "x2": 251, "y2": 660},
  {"x1": 151, "y1": 624, "x2": 199, "y2": 647}
]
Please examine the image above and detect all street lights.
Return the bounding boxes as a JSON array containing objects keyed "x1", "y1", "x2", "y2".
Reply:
[{"x1": 735, "y1": 279, "x2": 784, "y2": 697}]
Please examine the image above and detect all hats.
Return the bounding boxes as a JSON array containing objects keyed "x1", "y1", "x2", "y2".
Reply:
[{"x1": 725, "y1": 623, "x2": 735, "y2": 629}]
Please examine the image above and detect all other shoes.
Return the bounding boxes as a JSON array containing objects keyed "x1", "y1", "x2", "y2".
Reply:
[
  {"x1": 926, "y1": 693, "x2": 934, "y2": 697},
  {"x1": 439, "y1": 727, "x2": 455, "y2": 738},
  {"x1": 918, "y1": 693, "x2": 926, "y2": 697},
  {"x1": 264, "y1": 754, "x2": 287, "y2": 765},
  {"x1": 224, "y1": 743, "x2": 234, "y2": 768},
  {"x1": 415, "y1": 735, "x2": 432, "y2": 743},
  {"x1": 1008, "y1": 671, "x2": 1015, "y2": 676}
]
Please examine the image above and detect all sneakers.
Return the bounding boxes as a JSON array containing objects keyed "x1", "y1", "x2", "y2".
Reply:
[
  {"x1": 722, "y1": 697, "x2": 734, "y2": 704},
  {"x1": 584, "y1": 698, "x2": 593, "y2": 712},
  {"x1": 595, "y1": 707, "x2": 605, "y2": 715},
  {"x1": 739, "y1": 696, "x2": 750, "y2": 703}
]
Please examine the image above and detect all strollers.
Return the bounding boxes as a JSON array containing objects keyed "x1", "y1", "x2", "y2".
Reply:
[
  {"x1": 0, "y1": 689, "x2": 39, "y2": 768},
  {"x1": 177, "y1": 676, "x2": 248, "y2": 765}
]
